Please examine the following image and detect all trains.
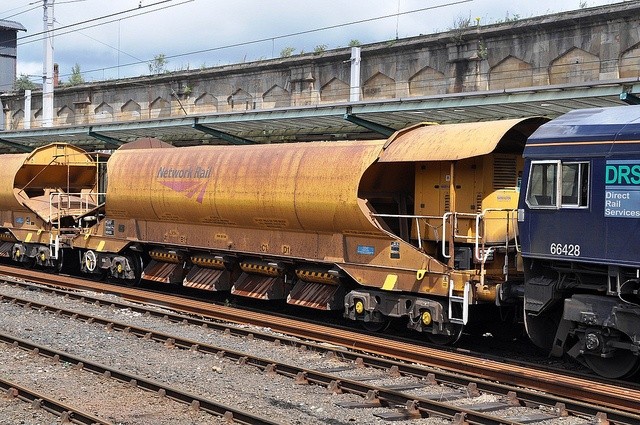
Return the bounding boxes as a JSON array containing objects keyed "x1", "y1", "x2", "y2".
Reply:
[{"x1": 1, "y1": 104, "x2": 640, "y2": 377}]
